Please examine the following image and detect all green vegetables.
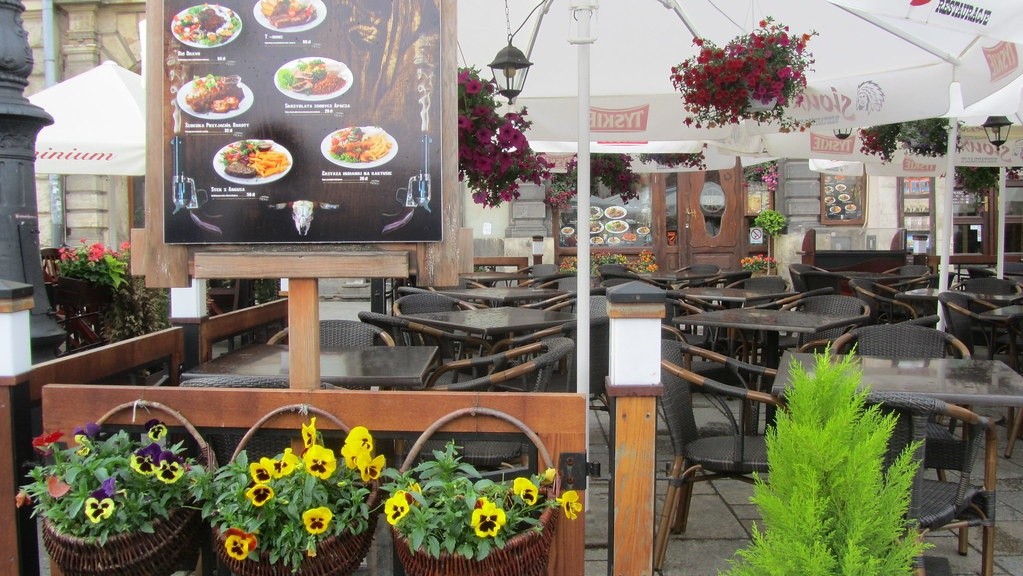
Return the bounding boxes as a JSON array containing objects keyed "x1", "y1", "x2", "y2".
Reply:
[
  {"x1": 193, "y1": 73, "x2": 221, "y2": 91},
  {"x1": 178, "y1": 5, "x2": 240, "y2": 46},
  {"x1": 277, "y1": 59, "x2": 327, "y2": 95},
  {"x1": 221, "y1": 141, "x2": 264, "y2": 166}
]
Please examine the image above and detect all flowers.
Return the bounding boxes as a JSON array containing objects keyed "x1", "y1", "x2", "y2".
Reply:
[
  {"x1": 744, "y1": 161, "x2": 778, "y2": 192},
  {"x1": 740, "y1": 254, "x2": 777, "y2": 271},
  {"x1": 568, "y1": 152, "x2": 641, "y2": 205},
  {"x1": 674, "y1": 21, "x2": 808, "y2": 129},
  {"x1": 14, "y1": 420, "x2": 186, "y2": 534},
  {"x1": 195, "y1": 417, "x2": 387, "y2": 559},
  {"x1": 458, "y1": 66, "x2": 557, "y2": 208},
  {"x1": 639, "y1": 152, "x2": 707, "y2": 172},
  {"x1": 859, "y1": 125, "x2": 900, "y2": 164},
  {"x1": 379, "y1": 443, "x2": 580, "y2": 552},
  {"x1": 55, "y1": 238, "x2": 174, "y2": 341}
]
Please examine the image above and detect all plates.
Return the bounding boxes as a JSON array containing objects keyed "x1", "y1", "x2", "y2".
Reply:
[
  {"x1": 590, "y1": 223, "x2": 604, "y2": 234},
  {"x1": 590, "y1": 237, "x2": 603, "y2": 244},
  {"x1": 825, "y1": 197, "x2": 836, "y2": 203},
  {"x1": 605, "y1": 220, "x2": 629, "y2": 234},
  {"x1": 321, "y1": 127, "x2": 398, "y2": 169},
  {"x1": 608, "y1": 237, "x2": 620, "y2": 244},
  {"x1": 274, "y1": 57, "x2": 354, "y2": 101},
  {"x1": 835, "y1": 184, "x2": 846, "y2": 191},
  {"x1": 590, "y1": 207, "x2": 604, "y2": 220},
  {"x1": 845, "y1": 205, "x2": 856, "y2": 211},
  {"x1": 605, "y1": 206, "x2": 627, "y2": 219},
  {"x1": 253, "y1": 0, "x2": 327, "y2": 33},
  {"x1": 171, "y1": 5, "x2": 242, "y2": 48},
  {"x1": 213, "y1": 140, "x2": 293, "y2": 185},
  {"x1": 825, "y1": 186, "x2": 834, "y2": 193},
  {"x1": 177, "y1": 78, "x2": 254, "y2": 119},
  {"x1": 830, "y1": 207, "x2": 841, "y2": 213},
  {"x1": 561, "y1": 227, "x2": 574, "y2": 234},
  {"x1": 637, "y1": 227, "x2": 650, "y2": 234},
  {"x1": 838, "y1": 194, "x2": 850, "y2": 201}
]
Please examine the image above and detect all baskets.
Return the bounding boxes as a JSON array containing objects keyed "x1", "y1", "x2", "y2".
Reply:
[
  {"x1": 212, "y1": 403, "x2": 381, "y2": 576},
  {"x1": 42, "y1": 400, "x2": 219, "y2": 576},
  {"x1": 390, "y1": 408, "x2": 559, "y2": 576}
]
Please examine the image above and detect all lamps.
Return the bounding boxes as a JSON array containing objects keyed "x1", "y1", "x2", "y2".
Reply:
[
  {"x1": 489, "y1": 33, "x2": 534, "y2": 104},
  {"x1": 982, "y1": 116, "x2": 1013, "y2": 150}
]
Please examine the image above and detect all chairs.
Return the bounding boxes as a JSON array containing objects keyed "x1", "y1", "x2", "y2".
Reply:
[{"x1": 265, "y1": 264, "x2": 1022, "y2": 574}]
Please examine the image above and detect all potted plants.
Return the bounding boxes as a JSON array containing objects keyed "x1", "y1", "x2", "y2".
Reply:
[
  {"x1": 753, "y1": 209, "x2": 789, "y2": 236},
  {"x1": 900, "y1": 119, "x2": 950, "y2": 156}
]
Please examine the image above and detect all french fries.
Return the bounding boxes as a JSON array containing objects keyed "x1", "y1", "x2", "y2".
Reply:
[
  {"x1": 261, "y1": 0, "x2": 281, "y2": 16},
  {"x1": 249, "y1": 151, "x2": 289, "y2": 177},
  {"x1": 365, "y1": 133, "x2": 392, "y2": 161}
]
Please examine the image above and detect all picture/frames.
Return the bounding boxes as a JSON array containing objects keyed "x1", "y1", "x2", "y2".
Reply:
[{"x1": 820, "y1": 167, "x2": 867, "y2": 227}]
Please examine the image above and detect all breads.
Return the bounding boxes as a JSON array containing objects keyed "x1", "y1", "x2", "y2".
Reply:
[{"x1": 225, "y1": 163, "x2": 256, "y2": 178}]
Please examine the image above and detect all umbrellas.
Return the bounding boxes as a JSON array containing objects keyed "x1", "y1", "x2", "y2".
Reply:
[
  {"x1": 139, "y1": 0, "x2": 1023, "y2": 513},
  {"x1": 25, "y1": 60, "x2": 146, "y2": 252}
]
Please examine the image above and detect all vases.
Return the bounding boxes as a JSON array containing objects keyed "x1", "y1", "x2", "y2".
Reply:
[{"x1": 593, "y1": 176, "x2": 619, "y2": 199}]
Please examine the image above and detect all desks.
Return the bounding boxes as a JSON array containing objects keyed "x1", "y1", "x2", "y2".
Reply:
[{"x1": 179, "y1": 271, "x2": 992, "y2": 436}]
